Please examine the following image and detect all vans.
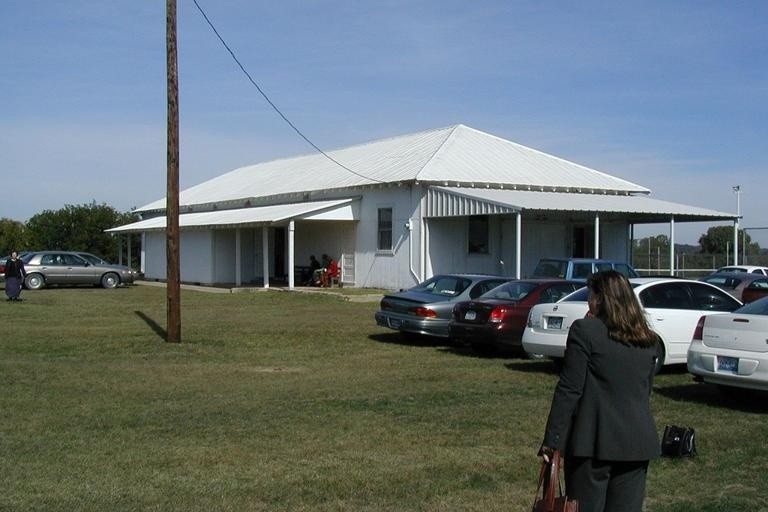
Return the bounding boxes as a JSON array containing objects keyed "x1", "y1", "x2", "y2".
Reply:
[{"x1": 528, "y1": 257, "x2": 640, "y2": 283}]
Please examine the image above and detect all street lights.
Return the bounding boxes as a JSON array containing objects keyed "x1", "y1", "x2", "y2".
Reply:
[{"x1": 732, "y1": 184, "x2": 743, "y2": 227}]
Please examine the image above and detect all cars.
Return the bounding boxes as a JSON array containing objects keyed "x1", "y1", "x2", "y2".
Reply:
[
  {"x1": 447, "y1": 276, "x2": 589, "y2": 354},
  {"x1": 686, "y1": 295, "x2": 768, "y2": 394},
  {"x1": 695, "y1": 272, "x2": 768, "y2": 304},
  {"x1": 0, "y1": 248, "x2": 139, "y2": 290},
  {"x1": 708, "y1": 264, "x2": 768, "y2": 288},
  {"x1": 522, "y1": 276, "x2": 747, "y2": 375},
  {"x1": 375, "y1": 272, "x2": 516, "y2": 338}
]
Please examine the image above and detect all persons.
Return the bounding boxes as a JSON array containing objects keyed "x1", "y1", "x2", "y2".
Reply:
[
  {"x1": 301, "y1": 255, "x2": 320, "y2": 286},
  {"x1": 537, "y1": 271, "x2": 666, "y2": 511},
  {"x1": 313, "y1": 254, "x2": 337, "y2": 287},
  {"x1": 4, "y1": 251, "x2": 27, "y2": 301}
]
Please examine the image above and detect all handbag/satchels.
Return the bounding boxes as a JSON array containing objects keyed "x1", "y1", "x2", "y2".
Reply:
[{"x1": 535, "y1": 497, "x2": 577, "y2": 512}]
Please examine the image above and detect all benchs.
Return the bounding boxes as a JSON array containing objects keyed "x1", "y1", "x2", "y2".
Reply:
[{"x1": 282, "y1": 265, "x2": 342, "y2": 289}]
